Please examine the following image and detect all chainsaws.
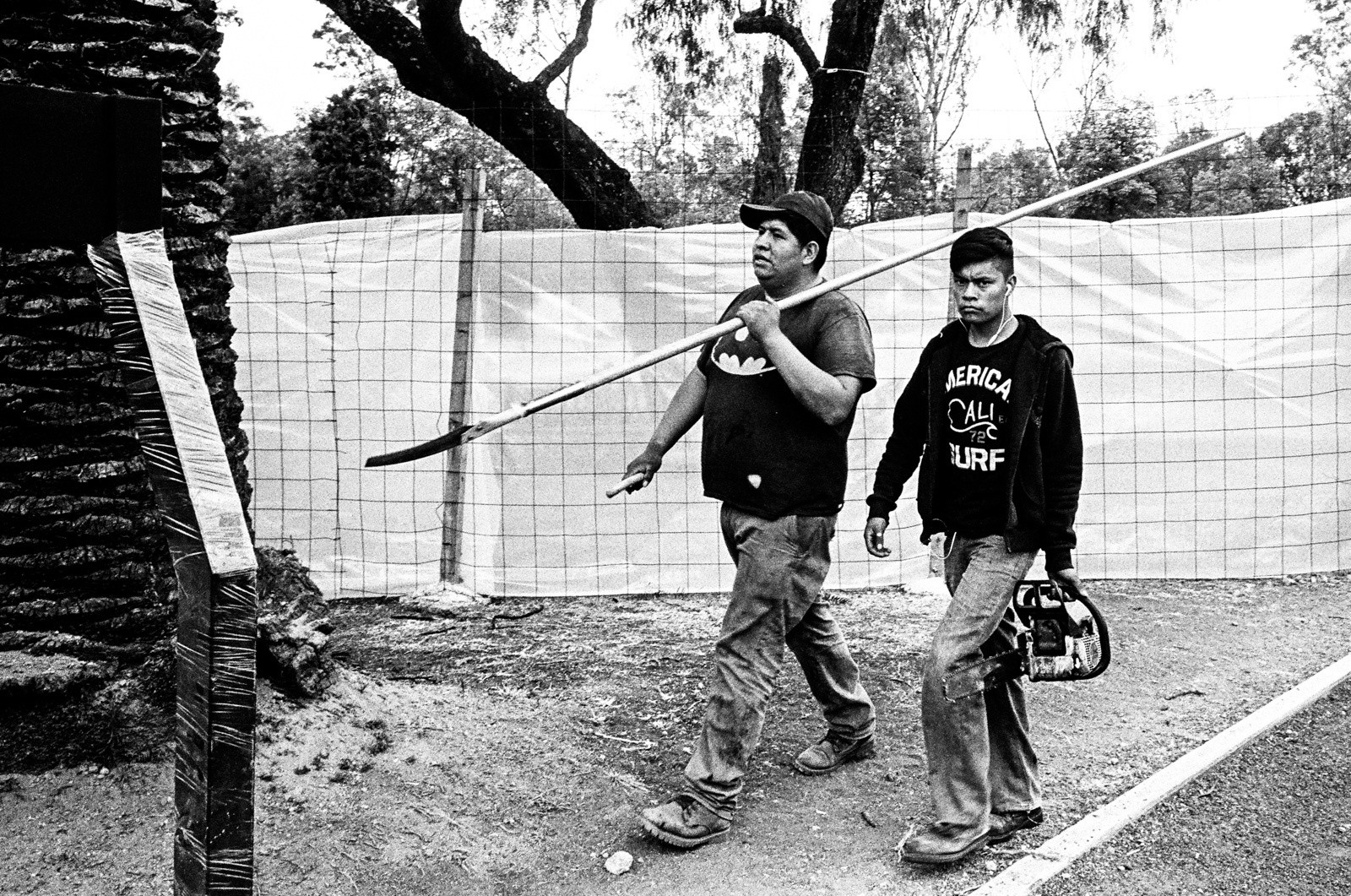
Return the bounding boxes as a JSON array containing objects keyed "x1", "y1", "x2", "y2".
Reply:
[{"x1": 943, "y1": 579, "x2": 1111, "y2": 703}]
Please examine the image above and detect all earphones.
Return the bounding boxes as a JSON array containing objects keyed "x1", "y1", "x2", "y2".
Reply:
[{"x1": 1005, "y1": 284, "x2": 1011, "y2": 297}]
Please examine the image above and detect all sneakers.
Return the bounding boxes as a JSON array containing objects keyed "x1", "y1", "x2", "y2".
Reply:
[
  {"x1": 904, "y1": 816, "x2": 990, "y2": 863},
  {"x1": 992, "y1": 808, "x2": 1045, "y2": 842},
  {"x1": 640, "y1": 797, "x2": 732, "y2": 846},
  {"x1": 795, "y1": 730, "x2": 879, "y2": 771}
]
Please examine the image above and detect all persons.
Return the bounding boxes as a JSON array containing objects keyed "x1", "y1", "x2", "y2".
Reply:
[
  {"x1": 865, "y1": 228, "x2": 1084, "y2": 862},
  {"x1": 620, "y1": 192, "x2": 877, "y2": 846}
]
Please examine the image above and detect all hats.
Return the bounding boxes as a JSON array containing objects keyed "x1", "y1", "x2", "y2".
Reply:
[{"x1": 739, "y1": 190, "x2": 833, "y2": 240}]
